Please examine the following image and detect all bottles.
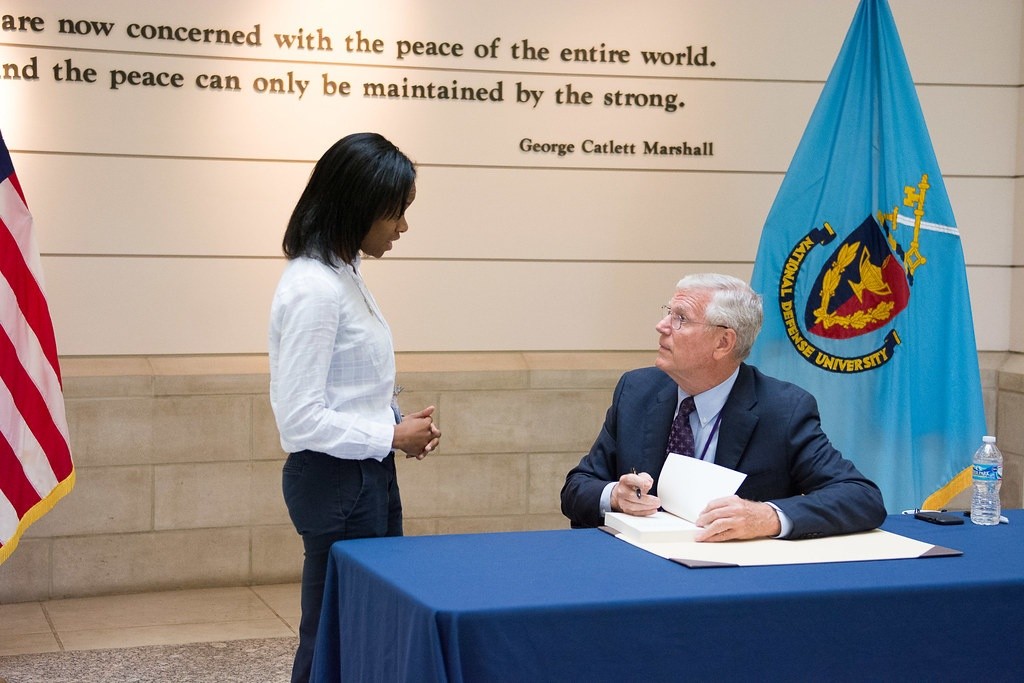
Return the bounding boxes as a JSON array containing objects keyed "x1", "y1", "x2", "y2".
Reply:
[{"x1": 970, "y1": 435, "x2": 1003, "y2": 526}]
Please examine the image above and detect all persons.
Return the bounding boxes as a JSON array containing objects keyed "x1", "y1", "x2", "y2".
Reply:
[
  {"x1": 266, "y1": 132, "x2": 442, "y2": 682},
  {"x1": 559, "y1": 273, "x2": 886, "y2": 548}
]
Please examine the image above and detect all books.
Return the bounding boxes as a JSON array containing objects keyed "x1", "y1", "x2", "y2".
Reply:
[{"x1": 596, "y1": 453, "x2": 747, "y2": 544}]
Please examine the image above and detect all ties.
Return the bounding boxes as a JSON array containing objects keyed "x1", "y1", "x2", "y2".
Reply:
[{"x1": 666, "y1": 397, "x2": 698, "y2": 459}]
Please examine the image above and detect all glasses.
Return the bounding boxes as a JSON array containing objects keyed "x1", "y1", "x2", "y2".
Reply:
[{"x1": 660, "y1": 304, "x2": 728, "y2": 331}]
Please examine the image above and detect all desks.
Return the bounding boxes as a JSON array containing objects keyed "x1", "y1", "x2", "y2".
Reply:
[{"x1": 308, "y1": 504, "x2": 1024, "y2": 683}]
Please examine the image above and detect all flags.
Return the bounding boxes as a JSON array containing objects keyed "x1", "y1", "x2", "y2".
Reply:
[
  {"x1": 747, "y1": 0, "x2": 987, "y2": 514},
  {"x1": 0, "y1": 137, "x2": 79, "y2": 582}
]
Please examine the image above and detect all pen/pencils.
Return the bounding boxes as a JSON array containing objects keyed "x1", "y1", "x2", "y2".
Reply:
[
  {"x1": 632, "y1": 468, "x2": 641, "y2": 499},
  {"x1": 963, "y1": 510, "x2": 1010, "y2": 524}
]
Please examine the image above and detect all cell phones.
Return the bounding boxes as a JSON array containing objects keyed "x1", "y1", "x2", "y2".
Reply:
[{"x1": 915, "y1": 511, "x2": 965, "y2": 525}]
